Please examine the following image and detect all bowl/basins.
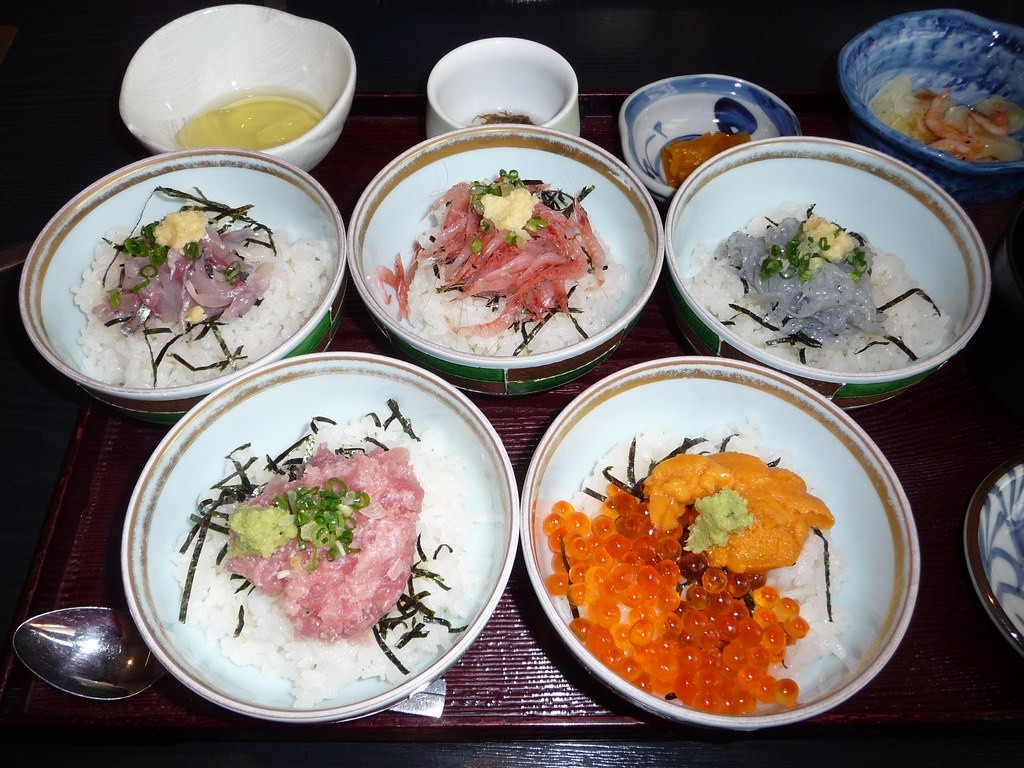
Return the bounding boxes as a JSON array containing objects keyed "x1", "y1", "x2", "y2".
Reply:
[
  {"x1": 618, "y1": 74, "x2": 803, "y2": 195},
  {"x1": 121, "y1": 350, "x2": 522, "y2": 722},
  {"x1": 839, "y1": 9, "x2": 1024, "y2": 204},
  {"x1": 345, "y1": 125, "x2": 665, "y2": 398},
  {"x1": 119, "y1": 4, "x2": 356, "y2": 172},
  {"x1": 964, "y1": 453, "x2": 1024, "y2": 655},
  {"x1": 18, "y1": 151, "x2": 347, "y2": 426},
  {"x1": 523, "y1": 358, "x2": 921, "y2": 727},
  {"x1": 667, "y1": 134, "x2": 992, "y2": 410}
]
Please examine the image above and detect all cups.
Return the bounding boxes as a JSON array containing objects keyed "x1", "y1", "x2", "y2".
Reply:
[{"x1": 427, "y1": 38, "x2": 581, "y2": 142}]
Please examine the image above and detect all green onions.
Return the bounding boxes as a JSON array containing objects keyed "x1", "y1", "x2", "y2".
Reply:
[
  {"x1": 109, "y1": 223, "x2": 242, "y2": 307},
  {"x1": 470, "y1": 168, "x2": 547, "y2": 254},
  {"x1": 276, "y1": 478, "x2": 369, "y2": 571},
  {"x1": 760, "y1": 231, "x2": 866, "y2": 281}
]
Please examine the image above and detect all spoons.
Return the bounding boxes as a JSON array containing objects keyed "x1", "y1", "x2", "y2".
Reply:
[{"x1": 14, "y1": 608, "x2": 447, "y2": 720}]
